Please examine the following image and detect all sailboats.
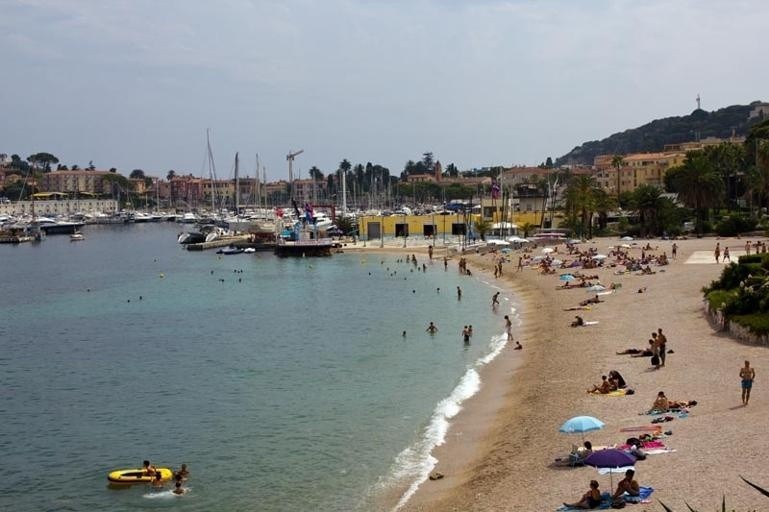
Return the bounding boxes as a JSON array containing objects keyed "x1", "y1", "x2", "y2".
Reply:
[
  {"x1": 177, "y1": 130, "x2": 411, "y2": 250},
  {"x1": 4, "y1": 163, "x2": 175, "y2": 243}
]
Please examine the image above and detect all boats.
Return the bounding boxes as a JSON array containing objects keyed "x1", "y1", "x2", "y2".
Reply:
[{"x1": 108, "y1": 468, "x2": 173, "y2": 486}]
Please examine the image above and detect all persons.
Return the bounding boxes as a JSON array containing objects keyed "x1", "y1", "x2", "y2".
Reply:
[
  {"x1": 608, "y1": 369, "x2": 629, "y2": 388},
  {"x1": 150, "y1": 471, "x2": 165, "y2": 486},
  {"x1": 653, "y1": 399, "x2": 698, "y2": 409},
  {"x1": 173, "y1": 470, "x2": 188, "y2": 481},
  {"x1": 586, "y1": 374, "x2": 610, "y2": 394},
  {"x1": 143, "y1": 458, "x2": 157, "y2": 475},
  {"x1": 738, "y1": 360, "x2": 756, "y2": 406},
  {"x1": 611, "y1": 468, "x2": 640, "y2": 498},
  {"x1": 562, "y1": 479, "x2": 601, "y2": 509},
  {"x1": 645, "y1": 390, "x2": 669, "y2": 414},
  {"x1": 179, "y1": 462, "x2": 190, "y2": 474},
  {"x1": 400, "y1": 234, "x2": 769, "y2": 371},
  {"x1": 173, "y1": 480, "x2": 184, "y2": 494},
  {"x1": 565, "y1": 439, "x2": 593, "y2": 466},
  {"x1": 596, "y1": 378, "x2": 617, "y2": 391}
]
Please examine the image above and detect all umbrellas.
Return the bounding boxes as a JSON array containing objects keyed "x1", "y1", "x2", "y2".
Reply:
[
  {"x1": 558, "y1": 414, "x2": 607, "y2": 449},
  {"x1": 583, "y1": 447, "x2": 638, "y2": 491}
]
